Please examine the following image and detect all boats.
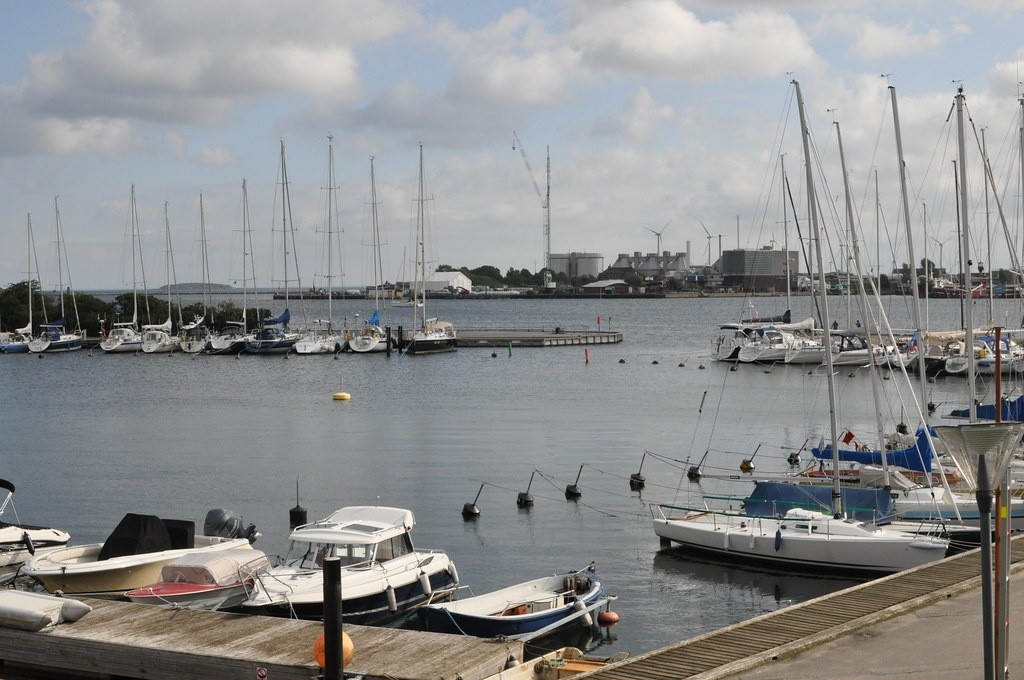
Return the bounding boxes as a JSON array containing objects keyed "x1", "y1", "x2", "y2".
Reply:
[
  {"x1": 18, "y1": 508, "x2": 263, "y2": 601},
  {"x1": 124, "y1": 547, "x2": 319, "y2": 613},
  {"x1": 419, "y1": 567, "x2": 603, "y2": 649},
  {"x1": 1, "y1": 477, "x2": 70, "y2": 591},
  {"x1": 244, "y1": 503, "x2": 461, "y2": 627}
]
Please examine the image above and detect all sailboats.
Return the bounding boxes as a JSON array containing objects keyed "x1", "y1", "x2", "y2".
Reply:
[
  {"x1": 644, "y1": 75, "x2": 1024, "y2": 571},
  {"x1": 5, "y1": 134, "x2": 458, "y2": 359}
]
